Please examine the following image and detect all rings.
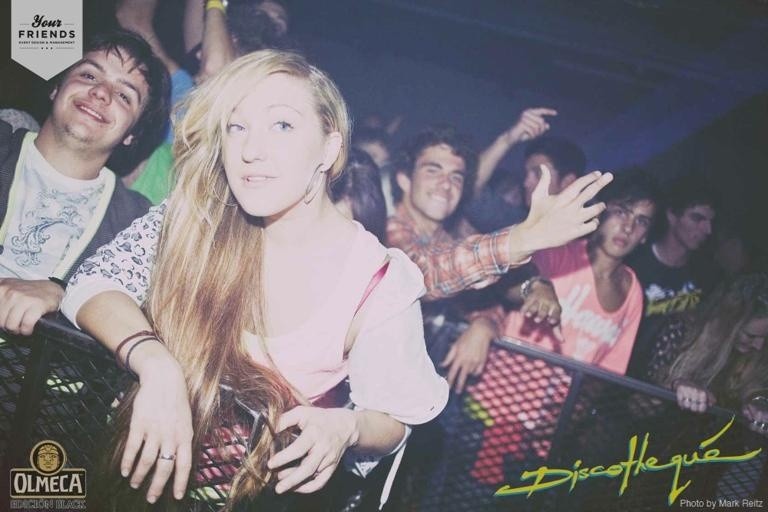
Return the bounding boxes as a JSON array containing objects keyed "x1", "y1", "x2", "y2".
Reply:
[{"x1": 158, "y1": 453, "x2": 176, "y2": 460}]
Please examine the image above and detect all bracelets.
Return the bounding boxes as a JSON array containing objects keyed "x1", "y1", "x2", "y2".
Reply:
[{"x1": 115, "y1": 331, "x2": 160, "y2": 381}]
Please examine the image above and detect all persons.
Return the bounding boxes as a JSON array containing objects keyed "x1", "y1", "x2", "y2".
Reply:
[
  {"x1": 60, "y1": 49, "x2": 450, "y2": 512},
  {"x1": 113, "y1": 1, "x2": 291, "y2": 207},
  {"x1": 326, "y1": 106, "x2": 768, "y2": 510},
  {"x1": 1, "y1": 29, "x2": 173, "y2": 512}
]
